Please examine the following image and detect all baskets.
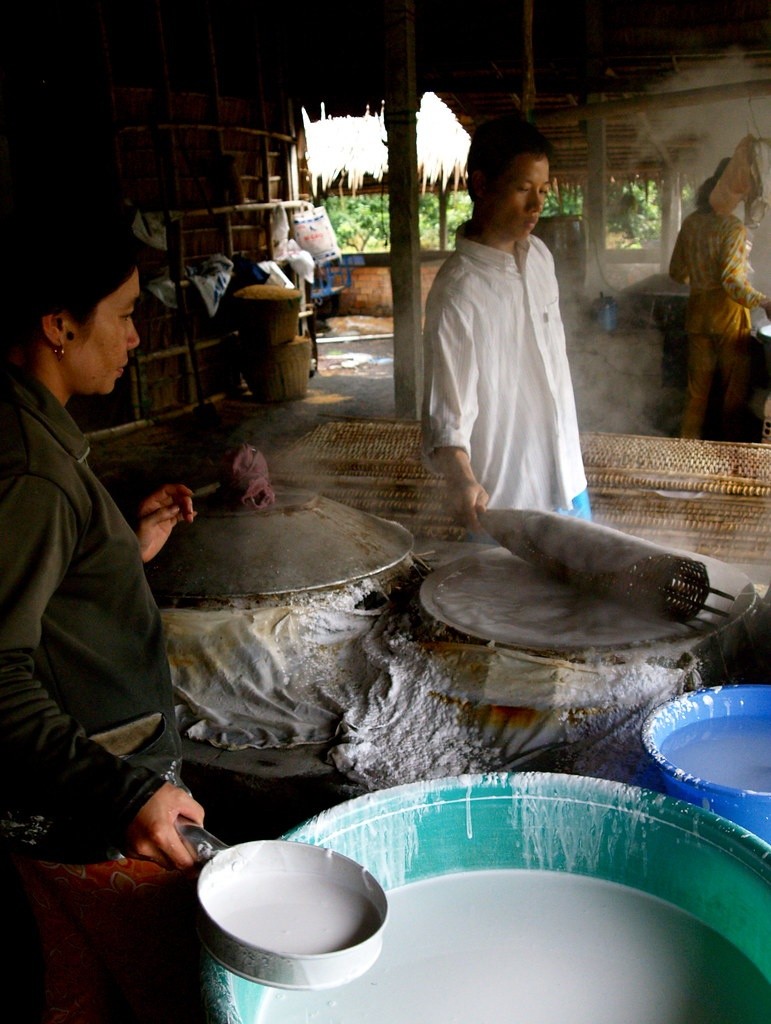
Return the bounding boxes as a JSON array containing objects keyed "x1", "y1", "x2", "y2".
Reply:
[{"x1": 232, "y1": 286, "x2": 312, "y2": 403}]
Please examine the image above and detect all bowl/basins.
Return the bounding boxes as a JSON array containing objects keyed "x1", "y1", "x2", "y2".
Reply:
[
  {"x1": 641, "y1": 683, "x2": 771, "y2": 843},
  {"x1": 198, "y1": 772, "x2": 771, "y2": 1024}
]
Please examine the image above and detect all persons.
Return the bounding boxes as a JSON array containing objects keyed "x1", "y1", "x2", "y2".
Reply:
[
  {"x1": 0, "y1": 228, "x2": 207, "y2": 1024},
  {"x1": 420, "y1": 115, "x2": 595, "y2": 544},
  {"x1": 668, "y1": 158, "x2": 771, "y2": 442}
]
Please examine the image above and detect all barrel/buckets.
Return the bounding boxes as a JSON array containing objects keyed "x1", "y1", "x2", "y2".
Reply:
[{"x1": 594, "y1": 305, "x2": 617, "y2": 333}]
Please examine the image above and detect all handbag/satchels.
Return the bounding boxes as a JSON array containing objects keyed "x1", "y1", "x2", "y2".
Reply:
[{"x1": 291, "y1": 201, "x2": 341, "y2": 265}]
